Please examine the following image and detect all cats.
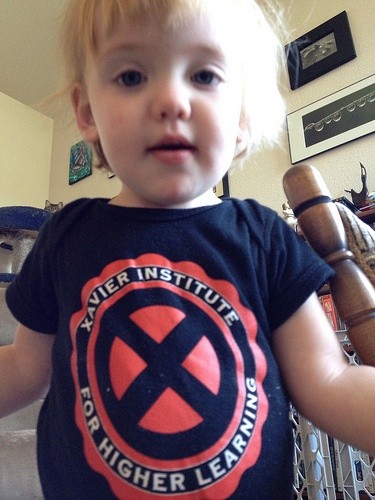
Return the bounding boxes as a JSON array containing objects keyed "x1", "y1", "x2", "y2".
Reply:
[{"x1": 43, "y1": 199, "x2": 64, "y2": 214}]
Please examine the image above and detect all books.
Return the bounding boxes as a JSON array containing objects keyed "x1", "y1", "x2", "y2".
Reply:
[{"x1": 319, "y1": 294, "x2": 348, "y2": 331}]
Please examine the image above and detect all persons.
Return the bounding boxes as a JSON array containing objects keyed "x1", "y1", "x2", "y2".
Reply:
[{"x1": 1, "y1": 0, "x2": 375, "y2": 500}]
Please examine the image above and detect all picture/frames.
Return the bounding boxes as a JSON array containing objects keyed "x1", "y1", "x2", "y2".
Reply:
[
  {"x1": 212, "y1": 172, "x2": 229, "y2": 198},
  {"x1": 286, "y1": 74, "x2": 375, "y2": 165},
  {"x1": 284, "y1": 10, "x2": 357, "y2": 91}
]
